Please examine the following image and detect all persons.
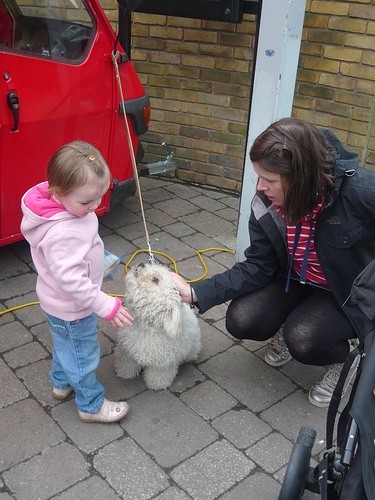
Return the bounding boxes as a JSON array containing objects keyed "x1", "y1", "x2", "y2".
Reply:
[
  {"x1": 166, "y1": 117, "x2": 374, "y2": 407},
  {"x1": 20, "y1": 141, "x2": 136, "y2": 423}
]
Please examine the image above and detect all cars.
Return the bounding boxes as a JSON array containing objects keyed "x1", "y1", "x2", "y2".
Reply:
[{"x1": 0, "y1": 0, "x2": 151, "y2": 247}]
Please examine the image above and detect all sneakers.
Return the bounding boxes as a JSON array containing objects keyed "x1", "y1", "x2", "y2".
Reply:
[
  {"x1": 308, "y1": 345, "x2": 361, "y2": 407},
  {"x1": 265, "y1": 323, "x2": 293, "y2": 367},
  {"x1": 78, "y1": 395, "x2": 129, "y2": 423},
  {"x1": 52, "y1": 386, "x2": 74, "y2": 398}
]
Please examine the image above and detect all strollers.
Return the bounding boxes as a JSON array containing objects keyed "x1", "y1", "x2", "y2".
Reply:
[{"x1": 276, "y1": 259, "x2": 375, "y2": 500}]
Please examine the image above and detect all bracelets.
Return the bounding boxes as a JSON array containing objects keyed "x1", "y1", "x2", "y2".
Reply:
[{"x1": 189, "y1": 285, "x2": 195, "y2": 309}]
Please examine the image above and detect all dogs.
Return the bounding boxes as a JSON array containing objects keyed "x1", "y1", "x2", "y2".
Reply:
[{"x1": 114, "y1": 262, "x2": 201, "y2": 392}]
